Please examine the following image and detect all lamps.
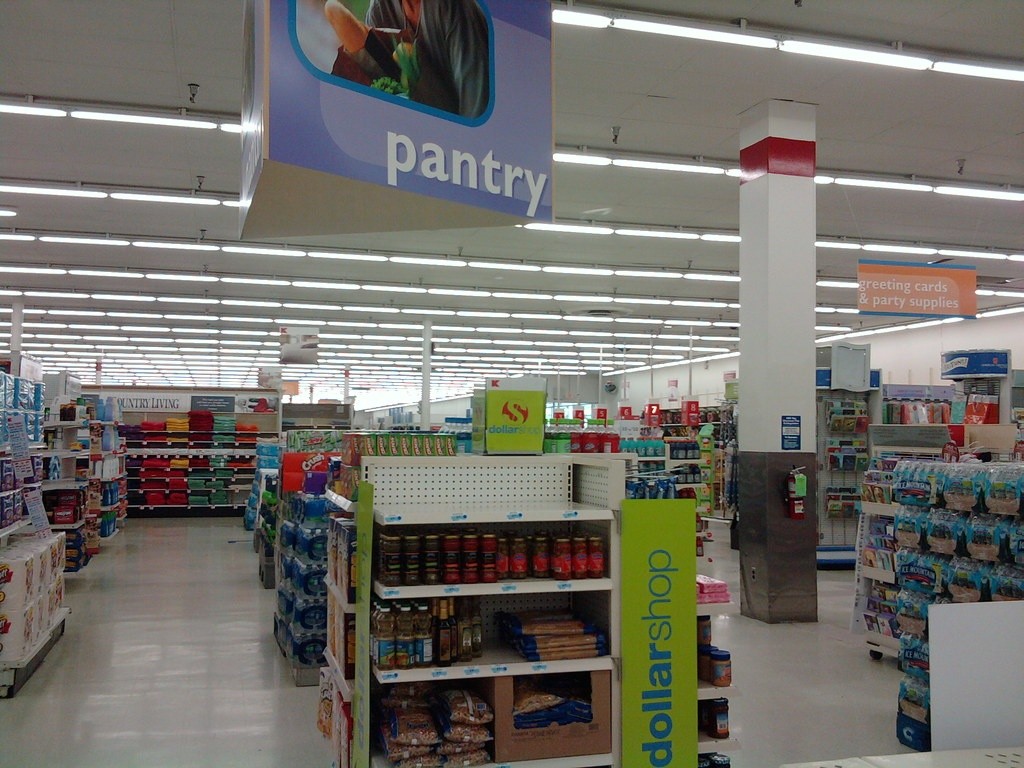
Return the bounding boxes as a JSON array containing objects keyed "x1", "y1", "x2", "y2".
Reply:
[{"x1": 1, "y1": 3, "x2": 1024, "y2": 386}]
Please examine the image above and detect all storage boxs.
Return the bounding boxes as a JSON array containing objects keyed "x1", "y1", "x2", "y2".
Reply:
[{"x1": 487, "y1": 670, "x2": 610, "y2": 762}]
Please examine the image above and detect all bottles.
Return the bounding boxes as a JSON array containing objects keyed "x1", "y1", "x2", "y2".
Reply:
[
  {"x1": 619, "y1": 437, "x2": 665, "y2": 457},
  {"x1": 272, "y1": 521, "x2": 327, "y2": 666},
  {"x1": 372, "y1": 533, "x2": 604, "y2": 583},
  {"x1": 544, "y1": 418, "x2": 619, "y2": 453},
  {"x1": 667, "y1": 438, "x2": 700, "y2": 459},
  {"x1": 369, "y1": 597, "x2": 483, "y2": 670},
  {"x1": 439, "y1": 417, "x2": 473, "y2": 452},
  {"x1": 671, "y1": 463, "x2": 701, "y2": 484}
]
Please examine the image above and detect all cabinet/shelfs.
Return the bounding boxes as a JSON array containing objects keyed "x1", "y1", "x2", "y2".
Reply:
[{"x1": 0, "y1": 373, "x2": 1024, "y2": 767}]
